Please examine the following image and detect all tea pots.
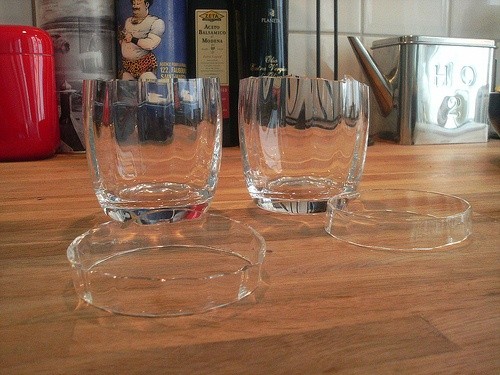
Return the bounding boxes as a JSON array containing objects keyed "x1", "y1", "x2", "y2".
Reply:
[{"x1": 346, "y1": 35, "x2": 498, "y2": 148}]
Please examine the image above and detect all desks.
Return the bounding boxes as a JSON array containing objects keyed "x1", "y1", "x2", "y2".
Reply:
[{"x1": 0, "y1": 136, "x2": 500, "y2": 375}]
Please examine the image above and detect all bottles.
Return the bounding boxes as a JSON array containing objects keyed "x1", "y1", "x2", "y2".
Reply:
[
  {"x1": 235, "y1": 1, "x2": 285, "y2": 130},
  {"x1": 114, "y1": 1, "x2": 191, "y2": 79},
  {"x1": 31, "y1": 0, "x2": 117, "y2": 155},
  {"x1": 187, "y1": 0, "x2": 239, "y2": 147},
  {"x1": 91, "y1": 80, "x2": 202, "y2": 146}
]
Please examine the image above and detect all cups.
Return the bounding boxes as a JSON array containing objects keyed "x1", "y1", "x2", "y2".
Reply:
[
  {"x1": 237, "y1": 76, "x2": 370, "y2": 217},
  {"x1": 81, "y1": 79, "x2": 223, "y2": 225}
]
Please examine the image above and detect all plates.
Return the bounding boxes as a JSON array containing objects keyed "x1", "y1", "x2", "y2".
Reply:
[
  {"x1": 66, "y1": 206, "x2": 266, "y2": 319},
  {"x1": 325, "y1": 188, "x2": 473, "y2": 251}
]
